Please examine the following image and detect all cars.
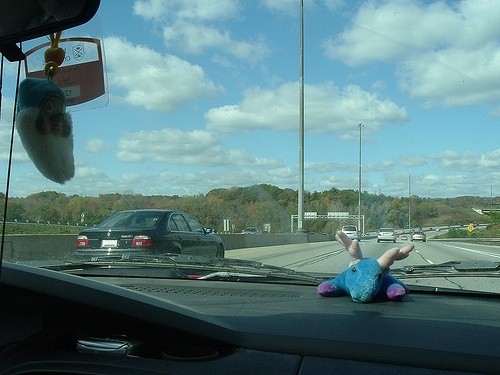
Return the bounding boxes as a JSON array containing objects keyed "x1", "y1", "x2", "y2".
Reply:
[
  {"x1": 401, "y1": 236, "x2": 408, "y2": 241},
  {"x1": 411, "y1": 231, "x2": 427, "y2": 242},
  {"x1": 376, "y1": 228, "x2": 398, "y2": 243},
  {"x1": 397, "y1": 228, "x2": 422, "y2": 235},
  {"x1": 241, "y1": 227, "x2": 263, "y2": 234},
  {"x1": 75, "y1": 208, "x2": 225, "y2": 260}
]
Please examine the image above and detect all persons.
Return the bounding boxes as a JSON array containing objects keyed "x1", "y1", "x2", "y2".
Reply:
[{"x1": 134, "y1": 214, "x2": 147, "y2": 227}]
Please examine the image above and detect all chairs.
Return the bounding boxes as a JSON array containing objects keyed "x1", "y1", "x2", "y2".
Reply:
[{"x1": 136, "y1": 215, "x2": 145, "y2": 225}]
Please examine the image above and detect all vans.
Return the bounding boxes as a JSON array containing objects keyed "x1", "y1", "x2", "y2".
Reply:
[{"x1": 342, "y1": 224, "x2": 358, "y2": 240}]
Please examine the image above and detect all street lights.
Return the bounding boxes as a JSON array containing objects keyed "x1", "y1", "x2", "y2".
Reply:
[
  {"x1": 407, "y1": 173, "x2": 413, "y2": 235},
  {"x1": 356, "y1": 122, "x2": 366, "y2": 241}
]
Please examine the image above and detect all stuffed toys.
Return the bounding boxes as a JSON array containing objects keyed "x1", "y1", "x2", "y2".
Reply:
[
  {"x1": 14, "y1": 74, "x2": 76, "y2": 183},
  {"x1": 317, "y1": 231, "x2": 415, "y2": 304}
]
